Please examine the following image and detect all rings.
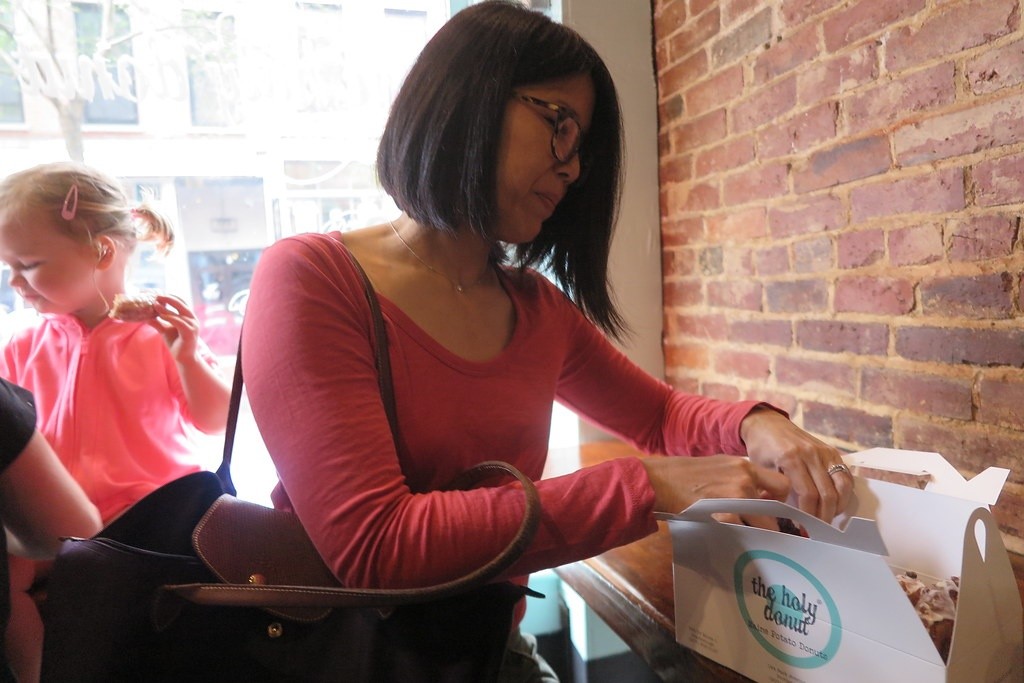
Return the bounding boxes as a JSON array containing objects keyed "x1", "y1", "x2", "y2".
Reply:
[{"x1": 829, "y1": 463, "x2": 850, "y2": 476}]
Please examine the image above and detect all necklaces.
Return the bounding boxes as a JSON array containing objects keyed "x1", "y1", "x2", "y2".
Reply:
[{"x1": 388, "y1": 220, "x2": 484, "y2": 294}]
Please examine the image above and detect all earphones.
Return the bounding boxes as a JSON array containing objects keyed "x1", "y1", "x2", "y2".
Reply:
[{"x1": 96, "y1": 241, "x2": 107, "y2": 254}]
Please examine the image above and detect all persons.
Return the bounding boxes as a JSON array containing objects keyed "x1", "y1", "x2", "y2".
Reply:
[
  {"x1": 0, "y1": 379, "x2": 103, "y2": 683},
  {"x1": 0, "y1": 162, "x2": 231, "y2": 526},
  {"x1": 239, "y1": 0, "x2": 858, "y2": 683}
]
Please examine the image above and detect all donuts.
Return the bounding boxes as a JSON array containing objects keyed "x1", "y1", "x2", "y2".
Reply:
[
  {"x1": 897, "y1": 569, "x2": 960, "y2": 664},
  {"x1": 107, "y1": 288, "x2": 166, "y2": 322}
]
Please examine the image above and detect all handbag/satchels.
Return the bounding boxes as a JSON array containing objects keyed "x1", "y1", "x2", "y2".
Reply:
[{"x1": 27, "y1": 242, "x2": 547, "y2": 683}]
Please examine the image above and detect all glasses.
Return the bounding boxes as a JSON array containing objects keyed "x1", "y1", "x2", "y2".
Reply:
[{"x1": 509, "y1": 90, "x2": 591, "y2": 166}]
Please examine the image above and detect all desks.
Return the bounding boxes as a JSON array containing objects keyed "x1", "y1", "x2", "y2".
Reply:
[{"x1": 556, "y1": 521, "x2": 753, "y2": 683}]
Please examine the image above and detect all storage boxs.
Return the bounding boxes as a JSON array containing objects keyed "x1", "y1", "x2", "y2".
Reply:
[{"x1": 670, "y1": 448, "x2": 1024, "y2": 683}]
